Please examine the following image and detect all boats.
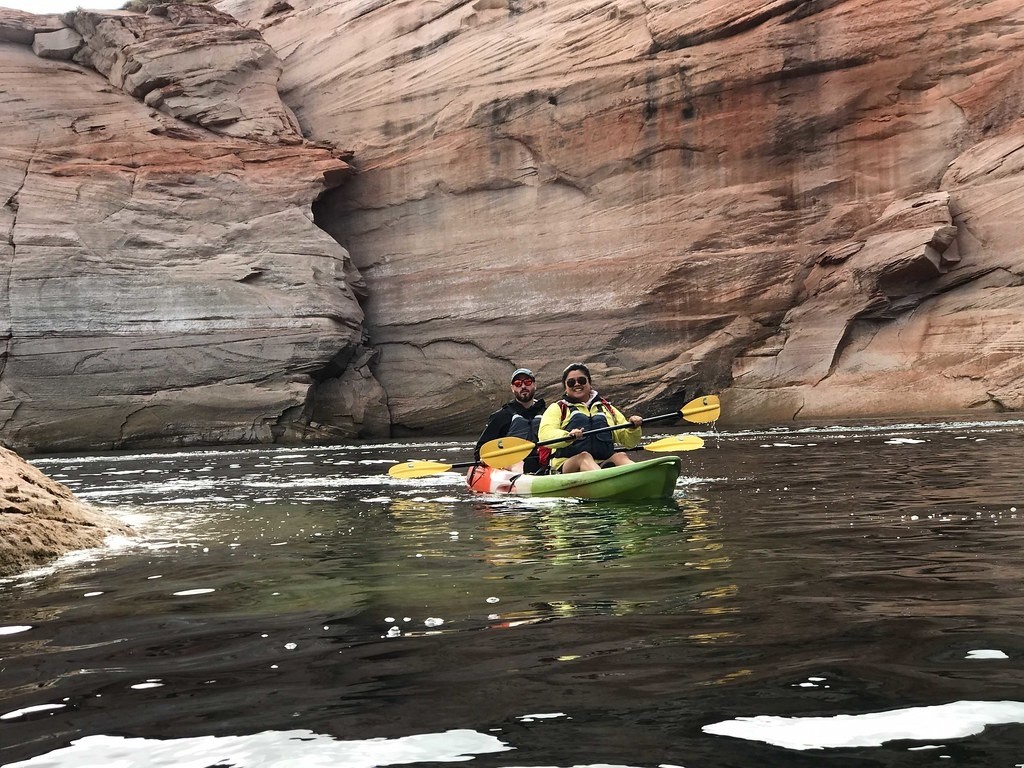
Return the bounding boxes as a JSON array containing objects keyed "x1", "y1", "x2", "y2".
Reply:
[{"x1": 466, "y1": 455, "x2": 682, "y2": 504}]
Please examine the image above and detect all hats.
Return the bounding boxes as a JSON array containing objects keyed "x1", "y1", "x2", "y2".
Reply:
[{"x1": 511, "y1": 368, "x2": 534, "y2": 383}]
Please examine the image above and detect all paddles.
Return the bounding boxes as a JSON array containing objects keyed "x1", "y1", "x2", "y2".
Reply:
[
  {"x1": 480, "y1": 393, "x2": 722, "y2": 468},
  {"x1": 388, "y1": 435, "x2": 704, "y2": 480}
]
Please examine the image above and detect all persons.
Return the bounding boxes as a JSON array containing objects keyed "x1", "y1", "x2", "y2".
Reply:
[
  {"x1": 474, "y1": 369, "x2": 552, "y2": 478},
  {"x1": 537, "y1": 364, "x2": 642, "y2": 474}
]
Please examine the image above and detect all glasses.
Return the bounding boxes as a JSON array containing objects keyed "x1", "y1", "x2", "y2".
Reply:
[
  {"x1": 567, "y1": 376, "x2": 588, "y2": 388},
  {"x1": 512, "y1": 379, "x2": 532, "y2": 387}
]
